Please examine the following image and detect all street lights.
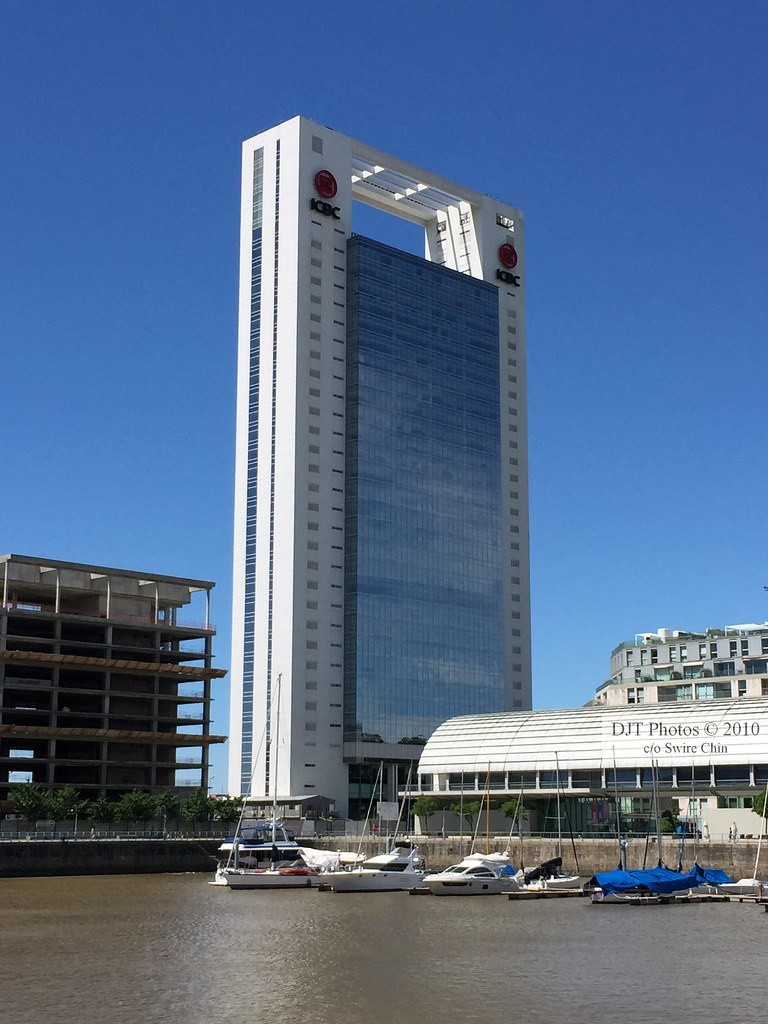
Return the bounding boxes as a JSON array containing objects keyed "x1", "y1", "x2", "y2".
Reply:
[
  {"x1": 160, "y1": 805, "x2": 168, "y2": 840},
  {"x1": 71, "y1": 804, "x2": 79, "y2": 841}
]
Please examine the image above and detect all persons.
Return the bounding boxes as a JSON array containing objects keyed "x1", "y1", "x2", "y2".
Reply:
[
  {"x1": 728, "y1": 827, "x2": 732, "y2": 842},
  {"x1": 732, "y1": 822, "x2": 737, "y2": 842}
]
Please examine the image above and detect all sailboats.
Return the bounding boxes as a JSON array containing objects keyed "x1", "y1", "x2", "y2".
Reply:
[{"x1": 206, "y1": 672, "x2": 768, "y2": 905}]
